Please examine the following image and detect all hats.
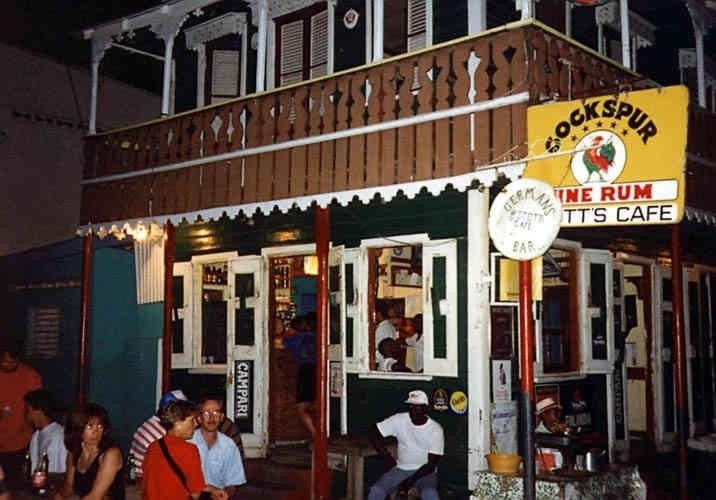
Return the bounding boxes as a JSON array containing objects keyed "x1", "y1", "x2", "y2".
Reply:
[
  {"x1": 404, "y1": 389, "x2": 429, "y2": 406},
  {"x1": 159, "y1": 390, "x2": 189, "y2": 410},
  {"x1": 534, "y1": 397, "x2": 564, "y2": 417}
]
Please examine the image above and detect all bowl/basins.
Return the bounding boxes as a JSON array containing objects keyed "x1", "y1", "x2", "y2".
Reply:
[{"x1": 482, "y1": 454, "x2": 522, "y2": 474}]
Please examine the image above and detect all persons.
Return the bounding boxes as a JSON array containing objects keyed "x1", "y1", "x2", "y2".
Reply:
[
  {"x1": 366, "y1": 389, "x2": 446, "y2": 500},
  {"x1": 129, "y1": 389, "x2": 248, "y2": 500},
  {"x1": 498, "y1": 362, "x2": 506, "y2": 385},
  {"x1": 282, "y1": 315, "x2": 318, "y2": 446},
  {"x1": 376, "y1": 337, "x2": 423, "y2": 374},
  {"x1": 273, "y1": 317, "x2": 289, "y2": 351},
  {"x1": 534, "y1": 397, "x2": 564, "y2": 434},
  {"x1": 398, "y1": 313, "x2": 422, "y2": 373},
  {"x1": 373, "y1": 306, "x2": 396, "y2": 370},
  {"x1": 2, "y1": 338, "x2": 43, "y2": 500},
  {"x1": 18, "y1": 388, "x2": 127, "y2": 500}
]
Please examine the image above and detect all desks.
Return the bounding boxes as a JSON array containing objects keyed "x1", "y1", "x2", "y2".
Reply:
[
  {"x1": 470, "y1": 460, "x2": 648, "y2": 500},
  {"x1": 320, "y1": 435, "x2": 395, "y2": 499}
]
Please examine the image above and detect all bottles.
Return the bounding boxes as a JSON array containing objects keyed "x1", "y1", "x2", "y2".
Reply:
[
  {"x1": 274, "y1": 301, "x2": 291, "y2": 329},
  {"x1": 272, "y1": 264, "x2": 289, "y2": 290},
  {"x1": 31, "y1": 464, "x2": 47, "y2": 496},
  {"x1": 202, "y1": 264, "x2": 228, "y2": 286}
]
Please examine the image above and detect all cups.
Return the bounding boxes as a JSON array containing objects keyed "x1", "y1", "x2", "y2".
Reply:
[
  {"x1": 536, "y1": 453, "x2": 555, "y2": 473},
  {"x1": 576, "y1": 455, "x2": 585, "y2": 471},
  {"x1": 587, "y1": 451, "x2": 604, "y2": 473}
]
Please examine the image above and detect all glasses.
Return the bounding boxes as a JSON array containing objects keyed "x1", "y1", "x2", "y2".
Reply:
[{"x1": 200, "y1": 407, "x2": 223, "y2": 417}]
